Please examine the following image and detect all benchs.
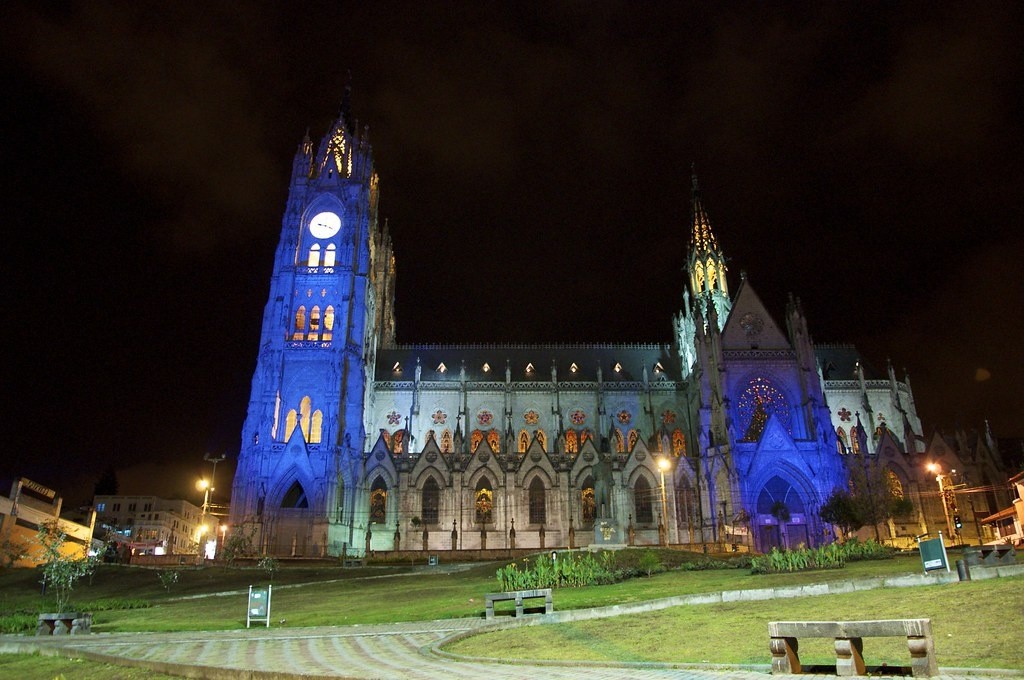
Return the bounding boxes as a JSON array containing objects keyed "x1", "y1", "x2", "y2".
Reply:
[
  {"x1": 484, "y1": 588, "x2": 554, "y2": 620},
  {"x1": 35, "y1": 612, "x2": 94, "y2": 637},
  {"x1": 767, "y1": 618, "x2": 940, "y2": 678},
  {"x1": 343, "y1": 558, "x2": 367, "y2": 567}
]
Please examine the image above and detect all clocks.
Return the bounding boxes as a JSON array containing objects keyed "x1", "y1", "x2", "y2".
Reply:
[{"x1": 309, "y1": 211, "x2": 342, "y2": 240}]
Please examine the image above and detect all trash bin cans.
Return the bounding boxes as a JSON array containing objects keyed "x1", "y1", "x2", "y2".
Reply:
[
  {"x1": 915, "y1": 529, "x2": 952, "y2": 572},
  {"x1": 247, "y1": 584, "x2": 271, "y2": 628}
]
